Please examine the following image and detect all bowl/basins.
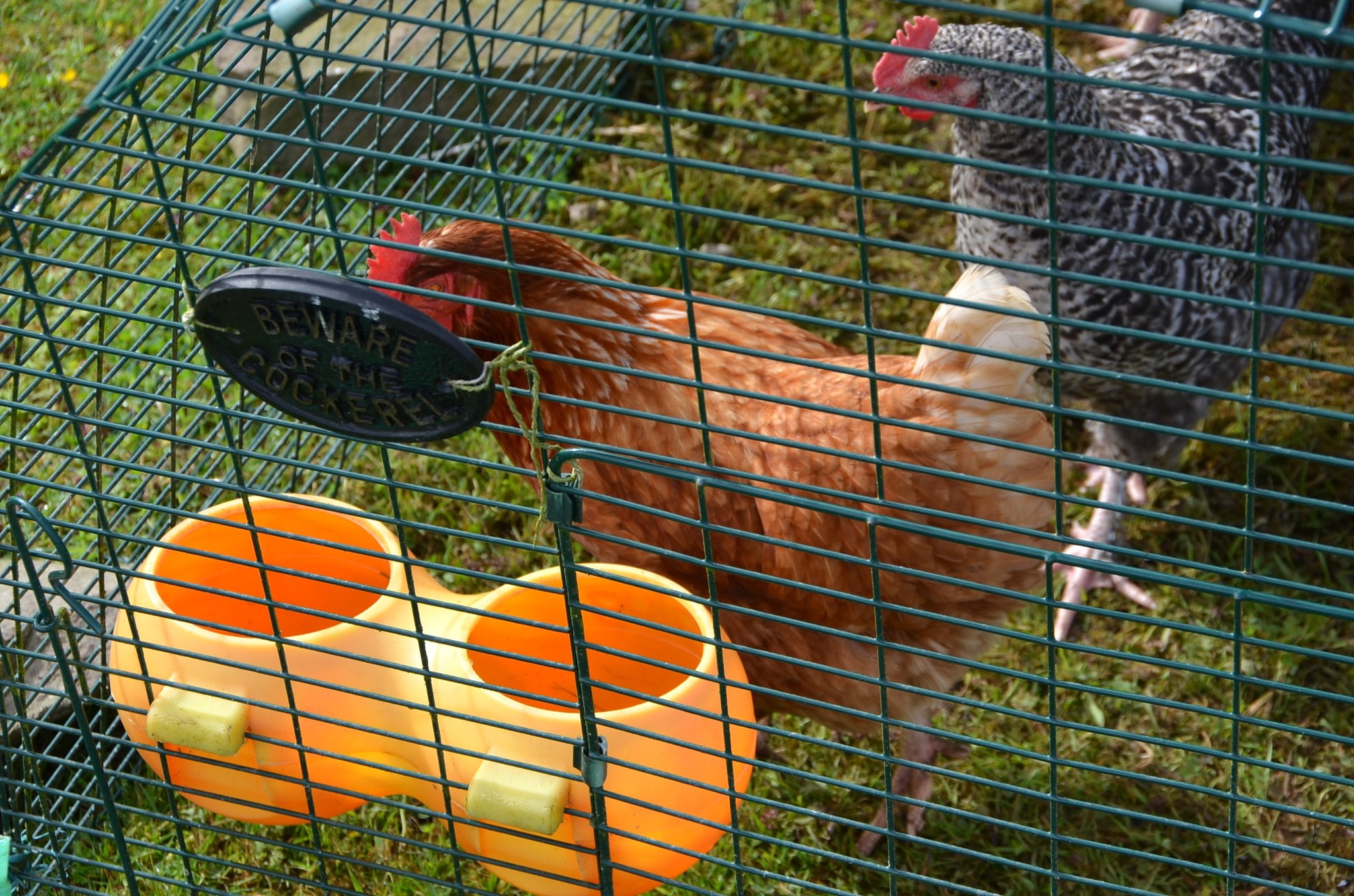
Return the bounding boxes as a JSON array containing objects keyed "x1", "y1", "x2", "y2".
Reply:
[{"x1": 110, "y1": 494, "x2": 756, "y2": 896}]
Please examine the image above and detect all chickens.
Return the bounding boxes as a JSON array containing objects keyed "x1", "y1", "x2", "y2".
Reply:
[
  {"x1": 365, "y1": 211, "x2": 1068, "y2": 861},
  {"x1": 862, "y1": 0, "x2": 1332, "y2": 644}
]
